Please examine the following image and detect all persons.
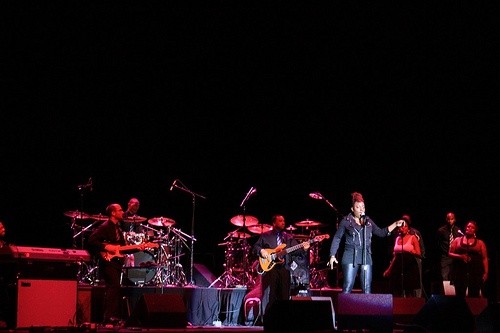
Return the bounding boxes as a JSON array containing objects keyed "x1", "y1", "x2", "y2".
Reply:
[
  {"x1": 383, "y1": 215, "x2": 424, "y2": 298},
  {"x1": 449, "y1": 221, "x2": 488, "y2": 298},
  {"x1": 327, "y1": 193, "x2": 405, "y2": 294},
  {"x1": 120, "y1": 198, "x2": 143, "y2": 233},
  {"x1": 89, "y1": 203, "x2": 145, "y2": 325},
  {"x1": 254, "y1": 215, "x2": 311, "y2": 302},
  {"x1": 436, "y1": 213, "x2": 464, "y2": 281}
]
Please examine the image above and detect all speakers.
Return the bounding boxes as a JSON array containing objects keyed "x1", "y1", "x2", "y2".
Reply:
[
  {"x1": 133, "y1": 291, "x2": 187, "y2": 327},
  {"x1": 334, "y1": 293, "x2": 394, "y2": 333},
  {"x1": 411, "y1": 296, "x2": 477, "y2": 333},
  {"x1": 263, "y1": 297, "x2": 336, "y2": 333},
  {"x1": 18, "y1": 279, "x2": 78, "y2": 328}
]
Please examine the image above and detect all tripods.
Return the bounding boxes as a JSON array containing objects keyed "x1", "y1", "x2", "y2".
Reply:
[
  {"x1": 204, "y1": 230, "x2": 334, "y2": 290},
  {"x1": 70, "y1": 217, "x2": 198, "y2": 285}
]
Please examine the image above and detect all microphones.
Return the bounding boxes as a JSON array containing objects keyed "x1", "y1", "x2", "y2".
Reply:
[
  {"x1": 309, "y1": 193, "x2": 323, "y2": 200},
  {"x1": 89, "y1": 179, "x2": 93, "y2": 191},
  {"x1": 246, "y1": 189, "x2": 256, "y2": 201},
  {"x1": 457, "y1": 230, "x2": 464, "y2": 235},
  {"x1": 361, "y1": 212, "x2": 370, "y2": 225},
  {"x1": 170, "y1": 181, "x2": 176, "y2": 190}
]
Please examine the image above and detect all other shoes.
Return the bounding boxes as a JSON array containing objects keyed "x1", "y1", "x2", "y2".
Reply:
[{"x1": 89, "y1": 318, "x2": 125, "y2": 329}]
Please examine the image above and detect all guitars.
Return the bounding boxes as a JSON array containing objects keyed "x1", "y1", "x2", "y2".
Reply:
[
  {"x1": 99, "y1": 242, "x2": 160, "y2": 262},
  {"x1": 258, "y1": 233, "x2": 331, "y2": 272}
]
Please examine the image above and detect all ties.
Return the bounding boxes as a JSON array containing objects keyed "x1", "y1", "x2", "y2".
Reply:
[
  {"x1": 276, "y1": 232, "x2": 284, "y2": 258},
  {"x1": 449, "y1": 228, "x2": 454, "y2": 248},
  {"x1": 115, "y1": 225, "x2": 120, "y2": 241}
]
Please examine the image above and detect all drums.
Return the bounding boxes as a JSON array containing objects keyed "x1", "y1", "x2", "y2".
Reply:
[
  {"x1": 306, "y1": 239, "x2": 328, "y2": 266},
  {"x1": 309, "y1": 266, "x2": 340, "y2": 290},
  {"x1": 225, "y1": 246, "x2": 256, "y2": 273},
  {"x1": 158, "y1": 242, "x2": 184, "y2": 271},
  {"x1": 114, "y1": 248, "x2": 158, "y2": 284}
]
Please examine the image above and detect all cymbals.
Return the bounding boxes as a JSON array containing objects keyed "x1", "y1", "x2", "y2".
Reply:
[
  {"x1": 295, "y1": 220, "x2": 322, "y2": 228},
  {"x1": 90, "y1": 214, "x2": 109, "y2": 223},
  {"x1": 120, "y1": 215, "x2": 147, "y2": 224},
  {"x1": 230, "y1": 215, "x2": 258, "y2": 227},
  {"x1": 248, "y1": 224, "x2": 273, "y2": 233},
  {"x1": 65, "y1": 211, "x2": 90, "y2": 221},
  {"x1": 148, "y1": 216, "x2": 176, "y2": 228},
  {"x1": 227, "y1": 233, "x2": 251, "y2": 240}
]
